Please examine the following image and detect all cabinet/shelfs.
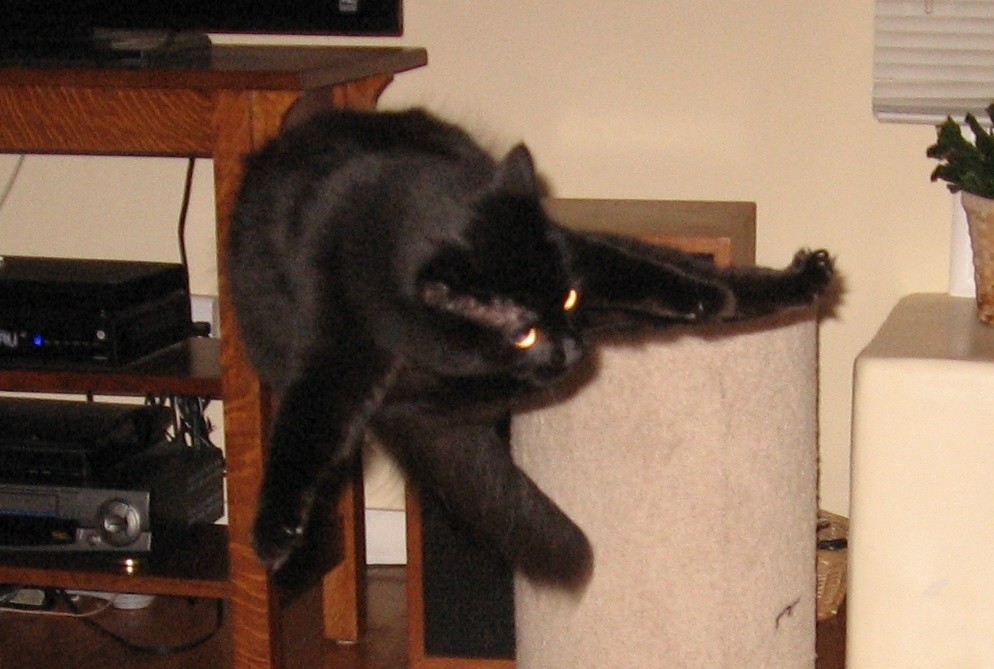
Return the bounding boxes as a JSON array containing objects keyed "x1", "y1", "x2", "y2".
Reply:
[{"x1": 845, "y1": 294, "x2": 994, "y2": 669}]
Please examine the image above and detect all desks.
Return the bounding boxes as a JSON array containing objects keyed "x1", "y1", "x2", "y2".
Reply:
[{"x1": 0, "y1": 46, "x2": 428, "y2": 669}]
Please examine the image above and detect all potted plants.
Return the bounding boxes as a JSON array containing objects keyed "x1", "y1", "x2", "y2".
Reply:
[{"x1": 927, "y1": 102, "x2": 994, "y2": 323}]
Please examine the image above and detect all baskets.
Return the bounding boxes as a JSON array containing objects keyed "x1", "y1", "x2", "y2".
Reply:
[{"x1": 816, "y1": 510, "x2": 850, "y2": 621}]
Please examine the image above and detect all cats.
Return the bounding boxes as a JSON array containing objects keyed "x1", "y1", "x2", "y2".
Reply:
[{"x1": 222, "y1": 104, "x2": 833, "y2": 593}]
